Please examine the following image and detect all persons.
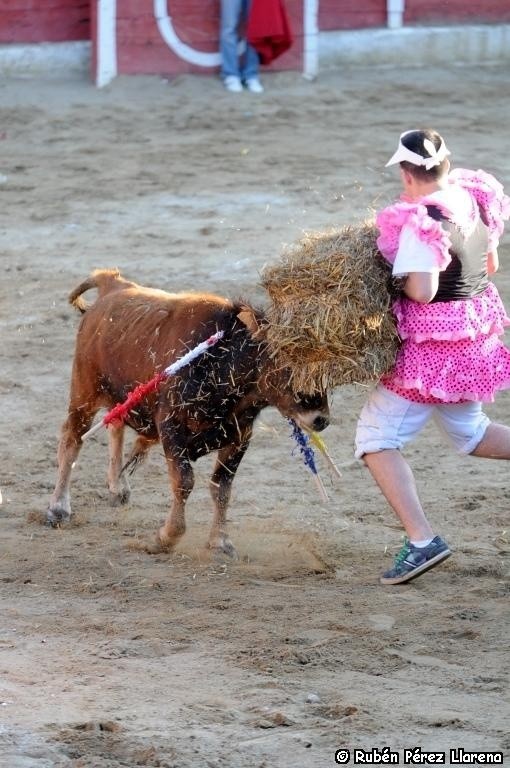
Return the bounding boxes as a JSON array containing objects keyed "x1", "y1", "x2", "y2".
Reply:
[
  {"x1": 218, "y1": 0, "x2": 266, "y2": 94},
  {"x1": 352, "y1": 127, "x2": 509, "y2": 585}
]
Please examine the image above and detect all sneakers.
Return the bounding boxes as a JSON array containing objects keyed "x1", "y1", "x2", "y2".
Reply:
[
  {"x1": 225, "y1": 75, "x2": 243, "y2": 94},
  {"x1": 245, "y1": 78, "x2": 265, "y2": 95},
  {"x1": 380, "y1": 534, "x2": 451, "y2": 585}
]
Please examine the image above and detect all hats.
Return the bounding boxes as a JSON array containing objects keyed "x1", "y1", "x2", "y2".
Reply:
[{"x1": 385, "y1": 129, "x2": 450, "y2": 169}]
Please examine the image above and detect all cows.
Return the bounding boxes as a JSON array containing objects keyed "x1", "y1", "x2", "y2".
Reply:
[{"x1": 45, "y1": 265, "x2": 331, "y2": 557}]
